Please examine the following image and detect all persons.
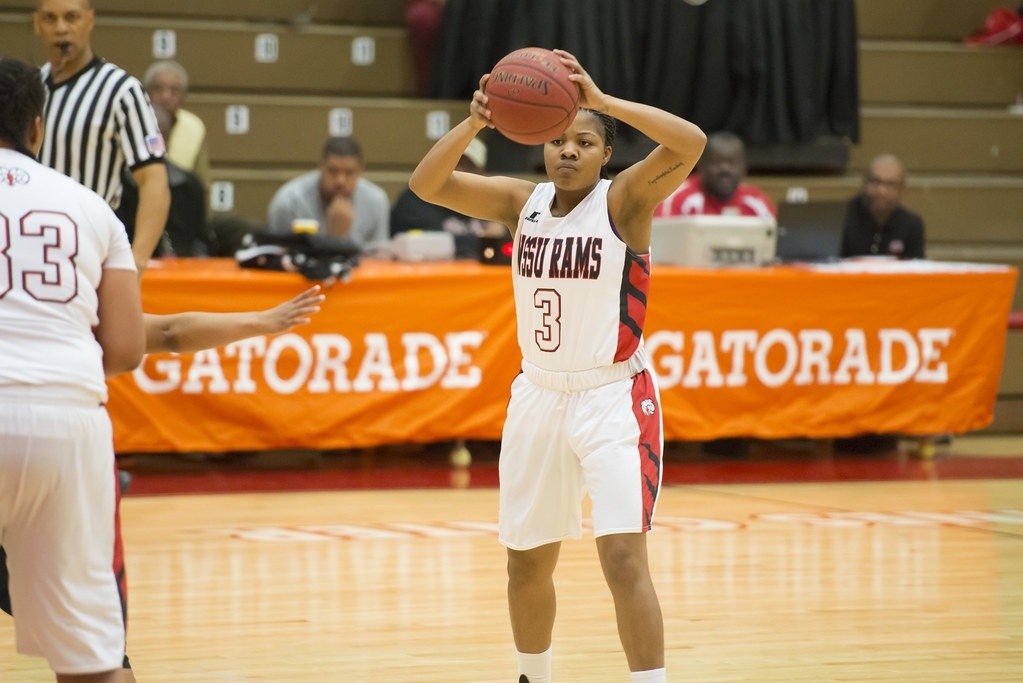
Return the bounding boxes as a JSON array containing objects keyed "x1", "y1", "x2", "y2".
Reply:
[
  {"x1": 33, "y1": 0, "x2": 171, "y2": 274},
  {"x1": 0, "y1": 58, "x2": 325, "y2": 683},
  {"x1": 391, "y1": 136, "x2": 487, "y2": 237},
  {"x1": 834, "y1": 153, "x2": 927, "y2": 458},
  {"x1": 142, "y1": 61, "x2": 211, "y2": 239},
  {"x1": 646, "y1": 132, "x2": 778, "y2": 221},
  {"x1": 407, "y1": 47, "x2": 707, "y2": 683},
  {"x1": 266, "y1": 137, "x2": 390, "y2": 250}
]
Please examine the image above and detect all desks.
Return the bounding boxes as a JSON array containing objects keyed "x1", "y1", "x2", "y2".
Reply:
[{"x1": 103, "y1": 253, "x2": 1018, "y2": 465}]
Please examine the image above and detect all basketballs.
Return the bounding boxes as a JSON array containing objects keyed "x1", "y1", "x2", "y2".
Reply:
[{"x1": 485, "y1": 47, "x2": 581, "y2": 145}]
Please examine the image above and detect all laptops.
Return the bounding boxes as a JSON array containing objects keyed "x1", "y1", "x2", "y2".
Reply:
[{"x1": 775, "y1": 198, "x2": 845, "y2": 263}]
